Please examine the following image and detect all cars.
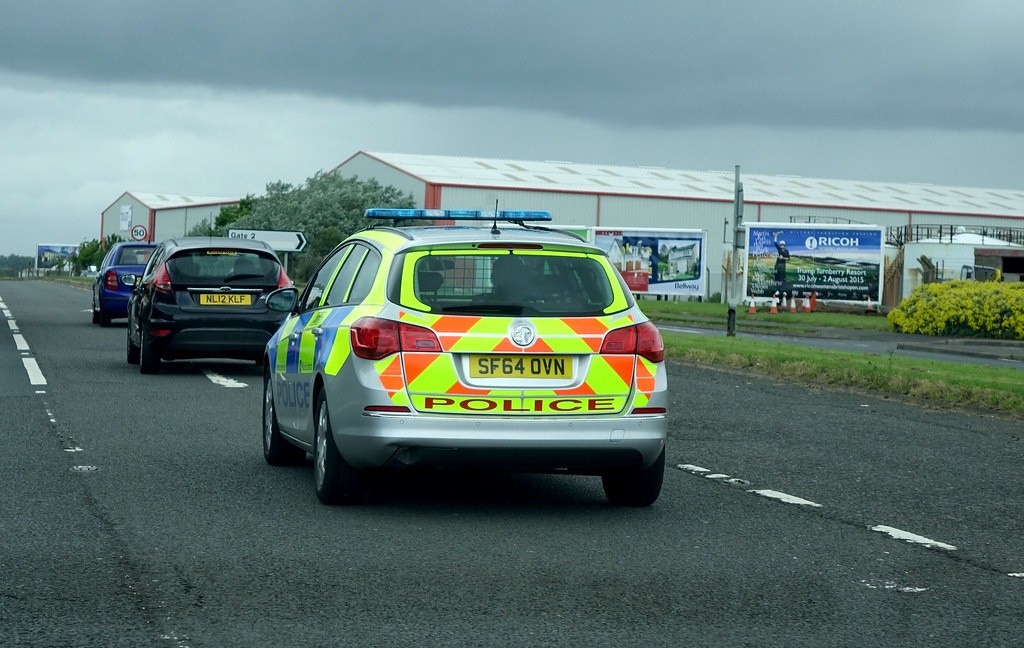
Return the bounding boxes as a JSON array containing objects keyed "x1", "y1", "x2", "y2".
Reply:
[
  {"x1": 119, "y1": 237, "x2": 298, "y2": 374},
  {"x1": 88, "y1": 241, "x2": 164, "y2": 328},
  {"x1": 263, "y1": 208, "x2": 668, "y2": 507}
]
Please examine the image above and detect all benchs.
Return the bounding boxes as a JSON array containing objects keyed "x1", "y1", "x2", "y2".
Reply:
[
  {"x1": 663, "y1": 292, "x2": 705, "y2": 303},
  {"x1": 632, "y1": 290, "x2": 664, "y2": 301}
]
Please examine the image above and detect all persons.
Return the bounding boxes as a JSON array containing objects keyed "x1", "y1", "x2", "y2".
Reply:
[
  {"x1": 490, "y1": 256, "x2": 522, "y2": 292},
  {"x1": 774, "y1": 234, "x2": 790, "y2": 283}
]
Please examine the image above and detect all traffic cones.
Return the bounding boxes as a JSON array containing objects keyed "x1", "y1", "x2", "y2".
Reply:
[
  {"x1": 803, "y1": 294, "x2": 811, "y2": 312},
  {"x1": 865, "y1": 295, "x2": 876, "y2": 315},
  {"x1": 746, "y1": 293, "x2": 758, "y2": 314},
  {"x1": 769, "y1": 294, "x2": 779, "y2": 314},
  {"x1": 789, "y1": 294, "x2": 798, "y2": 313},
  {"x1": 778, "y1": 292, "x2": 787, "y2": 313},
  {"x1": 809, "y1": 292, "x2": 818, "y2": 311},
  {"x1": 800, "y1": 292, "x2": 808, "y2": 312}
]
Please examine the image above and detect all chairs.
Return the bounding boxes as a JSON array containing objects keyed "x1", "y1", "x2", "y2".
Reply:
[
  {"x1": 121, "y1": 255, "x2": 138, "y2": 264},
  {"x1": 544, "y1": 274, "x2": 566, "y2": 303},
  {"x1": 233, "y1": 259, "x2": 256, "y2": 274},
  {"x1": 418, "y1": 272, "x2": 444, "y2": 302},
  {"x1": 174, "y1": 263, "x2": 200, "y2": 277}
]
